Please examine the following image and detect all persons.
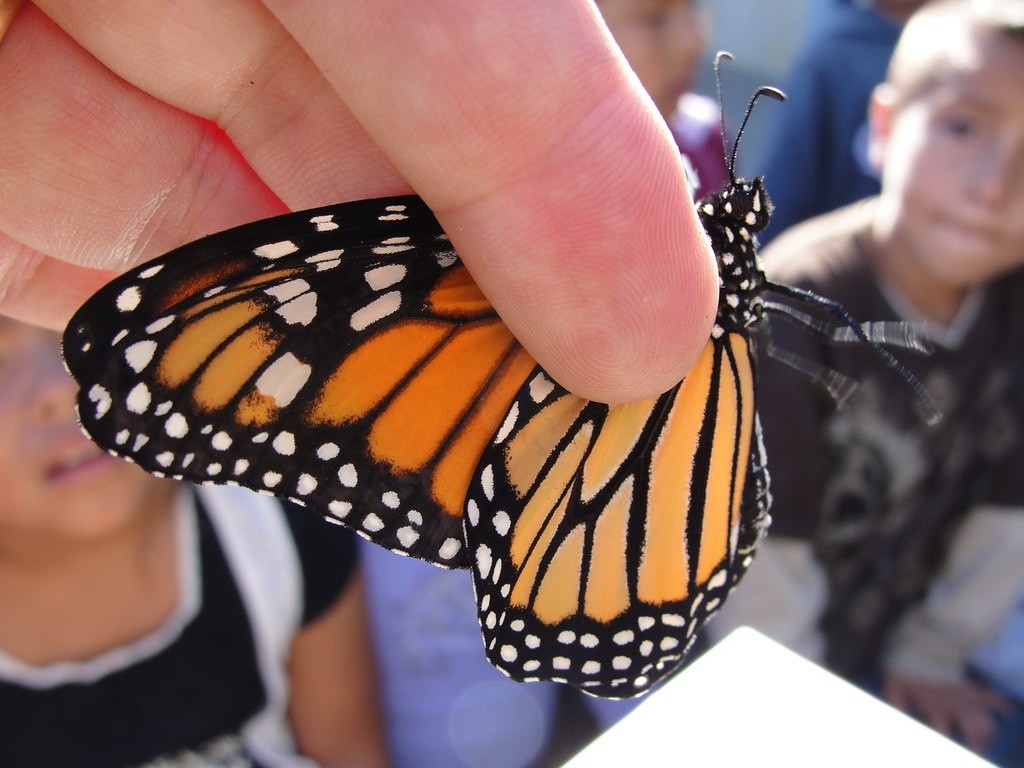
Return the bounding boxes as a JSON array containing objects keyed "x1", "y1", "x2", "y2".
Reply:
[
  {"x1": 0, "y1": 316, "x2": 392, "y2": 768},
  {"x1": 594, "y1": 1, "x2": 735, "y2": 258},
  {"x1": 705, "y1": 0, "x2": 1024, "y2": 763},
  {"x1": 739, "y1": 2, "x2": 923, "y2": 258},
  {"x1": 0, "y1": 1, "x2": 719, "y2": 403}
]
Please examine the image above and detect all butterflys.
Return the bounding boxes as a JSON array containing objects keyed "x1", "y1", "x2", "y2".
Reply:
[{"x1": 61, "y1": 52, "x2": 946, "y2": 700}]
